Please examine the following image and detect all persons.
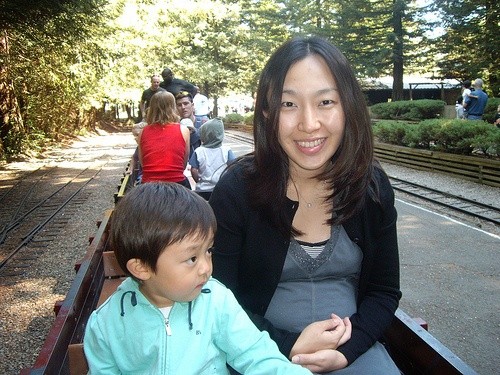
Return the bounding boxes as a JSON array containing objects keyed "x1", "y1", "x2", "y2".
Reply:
[
  {"x1": 456, "y1": 95, "x2": 468, "y2": 119},
  {"x1": 192, "y1": 86, "x2": 208, "y2": 115},
  {"x1": 208, "y1": 36, "x2": 403, "y2": 375},
  {"x1": 463, "y1": 78, "x2": 488, "y2": 120},
  {"x1": 461, "y1": 80, "x2": 478, "y2": 111},
  {"x1": 175, "y1": 92, "x2": 210, "y2": 191},
  {"x1": 82, "y1": 181, "x2": 313, "y2": 375},
  {"x1": 139, "y1": 76, "x2": 167, "y2": 122},
  {"x1": 138, "y1": 91, "x2": 192, "y2": 190},
  {"x1": 493, "y1": 104, "x2": 500, "y2": 128},
  {"x1": 159, "y1": 68, "x2": 197, "y2": 99},
  {"x1": 179, "y1": 117, "x2": 202, "y2": 162},
  {"x1": 189, "y1": 117, "x2": 235, "y2": 191}
]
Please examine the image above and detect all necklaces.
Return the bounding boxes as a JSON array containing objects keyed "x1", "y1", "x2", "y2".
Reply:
[{"x1": 287, "y1": 178, "x2": 327, "y2": 207}]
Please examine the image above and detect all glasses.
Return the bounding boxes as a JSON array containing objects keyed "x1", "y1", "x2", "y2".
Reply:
[{"x1": 175, "y1": 91, "x2": 193, "y2": 98}]
